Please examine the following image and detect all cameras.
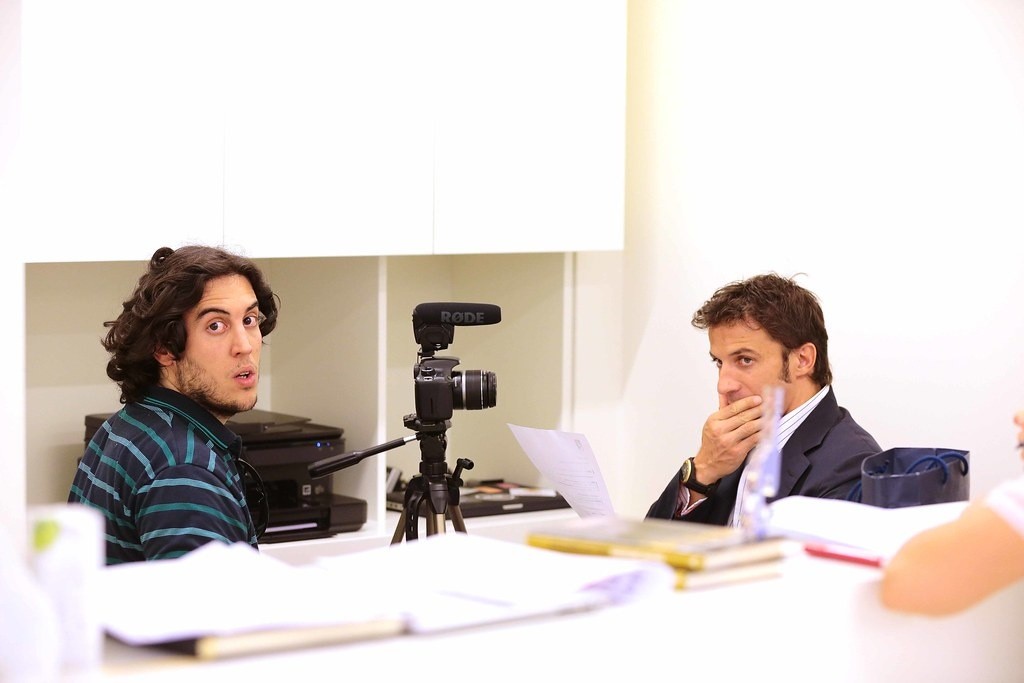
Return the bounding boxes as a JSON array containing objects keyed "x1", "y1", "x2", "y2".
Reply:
[{"x1": 413, "y1": 357, "x2": 498, "y2": 422}]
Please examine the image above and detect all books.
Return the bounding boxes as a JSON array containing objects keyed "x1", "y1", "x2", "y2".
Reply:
[
  {"x1": 101, "y1": 531, "x2": 647, "y2": 659},
  {"x1": 758, "y1": 497, "x2": 911, "y2": 568},
  {"x1": 527, "y1": 517, "x2": 790, "y2": 590}
]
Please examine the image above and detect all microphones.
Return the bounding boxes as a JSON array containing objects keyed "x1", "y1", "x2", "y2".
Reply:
[{"x1": 414, "y1": 303, "x2": 502, "y2": 326}]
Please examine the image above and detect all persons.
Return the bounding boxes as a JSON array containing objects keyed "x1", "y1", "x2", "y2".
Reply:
[
  {"x1": 68, "y1": 246, "x2": 281, "y2": 565},
  {"x1": 880, "y1": 411, "x2": 1024, "y2": 618},
  {"x1": 644, "y1": 275, "x2": 882, "y2": 527}
]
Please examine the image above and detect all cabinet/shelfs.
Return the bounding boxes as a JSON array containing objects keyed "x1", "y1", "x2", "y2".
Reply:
[
  {"x1": 15, "y1": 0, "x2": 434, "y2": 264},
  {"x1": 434, "y1": 0, "x2": 627, "y2": 255}
]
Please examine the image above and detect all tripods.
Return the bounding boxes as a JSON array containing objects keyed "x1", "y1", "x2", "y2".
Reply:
[{"x1": 307, "y1": 413, "x2": 474, "y2": 546}]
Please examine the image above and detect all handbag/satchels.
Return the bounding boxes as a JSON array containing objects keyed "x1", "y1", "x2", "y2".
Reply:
[{"x1": 845, "y1": 447, "x2": 970, "y2": 509}]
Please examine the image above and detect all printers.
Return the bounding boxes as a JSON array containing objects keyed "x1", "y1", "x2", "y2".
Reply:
[{"x1": 85, "y1": 407, "x2": 366, "y2": 545}]
